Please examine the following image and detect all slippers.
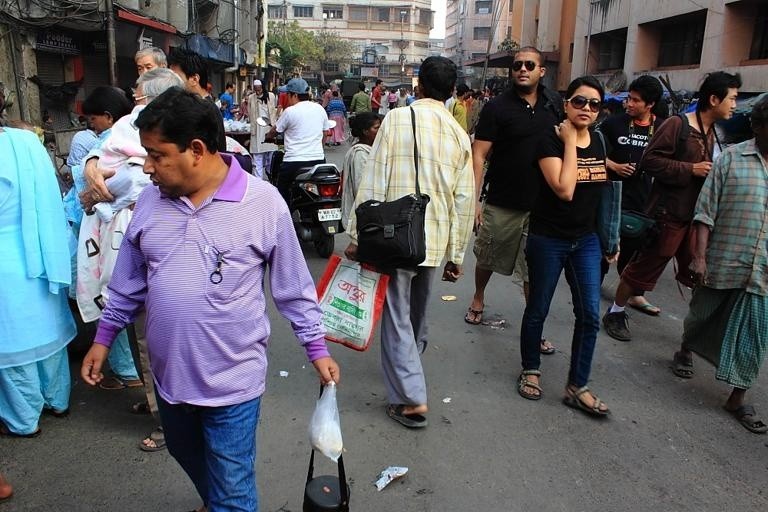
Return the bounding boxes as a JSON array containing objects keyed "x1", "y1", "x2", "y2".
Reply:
[
  {"x1": 629, "y1": 302, "x2": 660, "y2": 316},
  {"x1": 385, "y1": 401, "x2": 428, "y2": 428},
  {"x1": 139, "y1": 425, "x2": 168, "y2": 451},
  {"x1": 465, "y1": 303, "x2": 484, "y2": 325},
  {"x1": 672, "y1": 351, "x2": 695, "y2": 378},
  {"x1": 130, "y1": 399, "x2": 150, "y2": 415},
  {"x1": 0, "y1": 420, "x2": 41, "y2": 438},
  {"x1": 540, "y1": 336, "x2": 555, "y2": 354},
  {"x1": 42, "y1": 407, "x2": 71, "y2": 418},
  {"x1": 98, "y1": 375, "x2": 144, "y2": 390},
  {"x1": 722, "y1": 404, "x2": 767, "y2": 433}
]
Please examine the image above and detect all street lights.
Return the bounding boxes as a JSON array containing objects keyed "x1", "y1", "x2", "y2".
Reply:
[{"x1": 398, "y1": 10, "x2": 408, "y2": 87}]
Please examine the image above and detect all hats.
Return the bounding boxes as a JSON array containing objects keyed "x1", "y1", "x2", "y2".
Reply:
[
  {"x1": 253, "y1": 80, "x2": 262, "y2": 86},
  {"x1": 279, "y1": 78, "x2": 310, "y2": 95}
]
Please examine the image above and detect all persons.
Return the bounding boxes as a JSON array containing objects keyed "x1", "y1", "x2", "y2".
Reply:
[
  {"x1": 245, "y1": 74, "x2": 419, "y2": 230},
  {"x1": 344, "y1": 56, "x2": 476, "y2": 428},
  {"x1": 516, "y1": 76, "x2": 620, "y2": 417},
  {"x1": 59, "y1": 46, "x2": 250, "y2": 452},
  {"x1": 446, "y1": 46, "x2": 743, "y2": 354},
  {"x1": 0, "y1": 126, "x2": 78, "y2": 439},
  {"x1": 81, "y1": 86, "x2": 341, "y2": 512},
  {"x1": 672, "y1": 95, "x2": 768, "y2": 435}
]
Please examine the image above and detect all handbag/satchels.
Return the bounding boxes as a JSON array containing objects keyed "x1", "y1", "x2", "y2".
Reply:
[
  {"x1": 620, "y1": 209, "x2": 667, "y2": 252},
  {"x1": 316, "y1": 254, "x2": 390, "y2": 352},
  {"x1": 593, "y1": 128, "x2": 622, "y2": 257},
  {"x1": 355, "y1": 193, "x2": 430, "y2": 269}
]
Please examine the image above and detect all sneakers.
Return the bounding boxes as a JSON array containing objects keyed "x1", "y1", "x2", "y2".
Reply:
[{"x1": 602, "y1": 305, "x2": 633, "y2": 341}]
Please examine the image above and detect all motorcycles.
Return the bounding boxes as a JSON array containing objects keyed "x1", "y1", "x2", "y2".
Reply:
[{"x1": 257, "y1": 115, "x2": 350, "y2": 260}]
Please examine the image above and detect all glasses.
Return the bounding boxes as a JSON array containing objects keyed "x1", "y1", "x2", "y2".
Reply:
[
  {"x1": 133, "y1": 94, "x2": 157, "y2": 104},
  {"x1": 511, "y1": 61, "x2": 540, "y2": 71},
  {"x1": 566, "y1": 95, "x2": 601, "y2": 113}
]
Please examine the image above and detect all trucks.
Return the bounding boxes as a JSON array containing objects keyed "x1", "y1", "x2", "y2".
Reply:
[{"x1": 340, "y1": 77, "x2": 364, "y2": 109}]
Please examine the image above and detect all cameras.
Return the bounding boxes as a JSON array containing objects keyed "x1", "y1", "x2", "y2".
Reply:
[{"x1": 629, "y1": 163, "x2": 645, "y2": 180}]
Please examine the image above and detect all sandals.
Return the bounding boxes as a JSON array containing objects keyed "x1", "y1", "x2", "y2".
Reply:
[
  {"x1": 516, "y1": 368, "x2": 543, "y2": 400},
  {"x1": 563, "y1": 384, "x2": 611, "y2": 416}
]
